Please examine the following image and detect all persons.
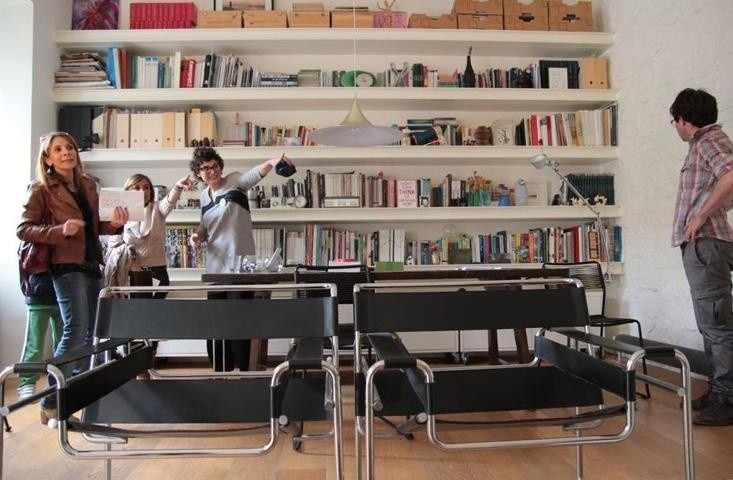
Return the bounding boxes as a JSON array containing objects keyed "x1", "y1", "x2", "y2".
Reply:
[
  {"x1": 669, "y1": 88, "x2": 733, "y2": 426},
  {"x1": 110, "y1": 172, "x2": 194, "y2": 380},
  {"x1": 19, "y1": 179, "x2": 66, "y2": 403},
  {"x1": 17, "y1": 130, "x2": 129, "y2": 427},
  {"x1": 188, "y1": 148, "x2": 291, "y2": 372}
]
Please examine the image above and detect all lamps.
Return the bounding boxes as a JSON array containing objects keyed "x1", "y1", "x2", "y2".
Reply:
[
  {"x1": 530, "y1": 155, "x2": 617, "y2": 283},
  {"x1": 306, "y1": 0, "x2": 404, "y2": 149}
]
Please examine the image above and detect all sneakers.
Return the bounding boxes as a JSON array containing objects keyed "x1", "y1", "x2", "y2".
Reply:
[
  {"x1": 690, "y1": 403, "x2": 733, "y2": 427},
  {"x1": 679, "y1": 397, "x2": 708, "y2": 409},
  {"x1": 19, "y1": 385, "x2": 36, "y2": 402}
]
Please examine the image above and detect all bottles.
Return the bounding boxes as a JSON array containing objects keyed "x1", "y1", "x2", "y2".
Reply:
[
  {"x1": 464, "y1": 56, "x2": 476, "y2": 88},
  {"x1": 412, "y1": 63, "x2": 425, "y2": 88}
]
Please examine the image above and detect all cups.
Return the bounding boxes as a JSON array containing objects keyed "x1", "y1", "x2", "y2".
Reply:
[{"x1": 228, "y1": 255, "x2": 242, "y2": 273}]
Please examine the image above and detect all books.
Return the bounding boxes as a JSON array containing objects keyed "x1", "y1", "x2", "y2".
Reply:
[
  {"x1": 59, "y1": 104, "x2": 315, "y2": 151},
  {"x1": 162, "y1": 227, "x2": 206, "y2": 270},
  {"x1": 318, "y1": 226, "x2": 408, "y2": 264},
  {"x1": 374, "y1": 52, "x2": 609, "y2": 90},
  {"x1": 52, "y1": 46, "x2": 318, "y2": 87},
  {"x1": 555, "y1": 170, "x2": 615, "y2": 204},
  {"x1": 511, "y1": 226, "x2": 622, "y2": 264},
  {"x1": 256, "y1": 169, "x2": 470, "y2": 208},
  {"x1": 406, "y1": 239, "x2": 443, "y2": 264},
  {"x1": 388, "y1": 106, "x2": 617, "y2": 146},
  {"x1": 441, "y1": 230, "x2": 512, "y2": 264},
  {"x1": 249, "y1": 225, "x2": 313, "y2": 270},
  {"x1": 128, "y1": 3, "x2": 197, "y2": 30}
]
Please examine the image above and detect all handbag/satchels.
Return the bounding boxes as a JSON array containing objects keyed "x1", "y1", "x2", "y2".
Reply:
[{"x1": 20, "y1": 183, "x2": 50, "y2": 273}]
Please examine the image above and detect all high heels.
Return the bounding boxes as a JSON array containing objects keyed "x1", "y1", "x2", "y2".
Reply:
[{"x1": 41, "y1": 409, "x2": 79, "y2": 425}]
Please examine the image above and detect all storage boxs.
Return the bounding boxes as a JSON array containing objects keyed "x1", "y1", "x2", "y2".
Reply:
[
  {"x1": 503, "y1": 0, "x2": 548, "y2": 31},
  {"x1": 456, "y1": 0, "x2": 503, "y2": 30},
  {"x1": 548, "y1": 0, "x2": 594, "y2": 32}
]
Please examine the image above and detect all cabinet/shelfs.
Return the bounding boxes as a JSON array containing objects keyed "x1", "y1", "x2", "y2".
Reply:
[
  {"x1": 77, "y1": 151, "x2": 620, "y2": 205},
  {"x1": 50, "y1": 30, "x2": 619, "y2": 90},
  {"x1": 161, "y1": 209, "x2": 624, "y2": 281},
  {"x1": 54, "y1": 88, "x2": 615, "y2": 146}
]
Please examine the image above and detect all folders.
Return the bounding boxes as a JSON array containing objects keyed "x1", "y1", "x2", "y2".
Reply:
[
  {"x1": 131, "y1": 106, "x2": 143, "y2": 151},
  {"x1": 174, "y1": 111, "x2": 186, "y2": 149},
  {"x1": 143, "y1": 109, "x2": 162, "y2": 149},
  {"x1": 582, "y1": 57, "x2": 596, "y2": 89},
  {"x1": 160, "y1": 111, "x2": 174, "y2": 147},
  {"x1": 107, "y1": 46, "x2": 128, "y2": 87},
  {"x1": 200, "y1": 104, "x2": 216, "y2": 147},
  {"x1": 116, "y1": 105, "x2": 129, "y2": 150},
  {"x1": 188, "y1": 103, "x2": 200, "y2": 147},
  {"x1": 596, "y1": 59, "x2": 608, "y2": 88}
]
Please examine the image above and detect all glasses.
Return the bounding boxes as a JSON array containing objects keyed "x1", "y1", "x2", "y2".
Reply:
[
  {"x1": 669, "y1": 117, "x2": 680, "y2": 124},
  {"x1": 198, "y1": 163, "x2": 222, "y2": 170}
]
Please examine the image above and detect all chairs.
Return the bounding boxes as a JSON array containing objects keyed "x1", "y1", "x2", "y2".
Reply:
[
  {"x1": 542, "y1": 261, "x2": 651, "y2": 399},
  {"x1": 354, "y1": 278, "x2": 646, "y2": 480},
  {"x1": 295, "y1": 265, "x2": 380, "y2": 376},
  {"x1": 1, "y1": 285, "x2": 343, "y2": 479}
]
